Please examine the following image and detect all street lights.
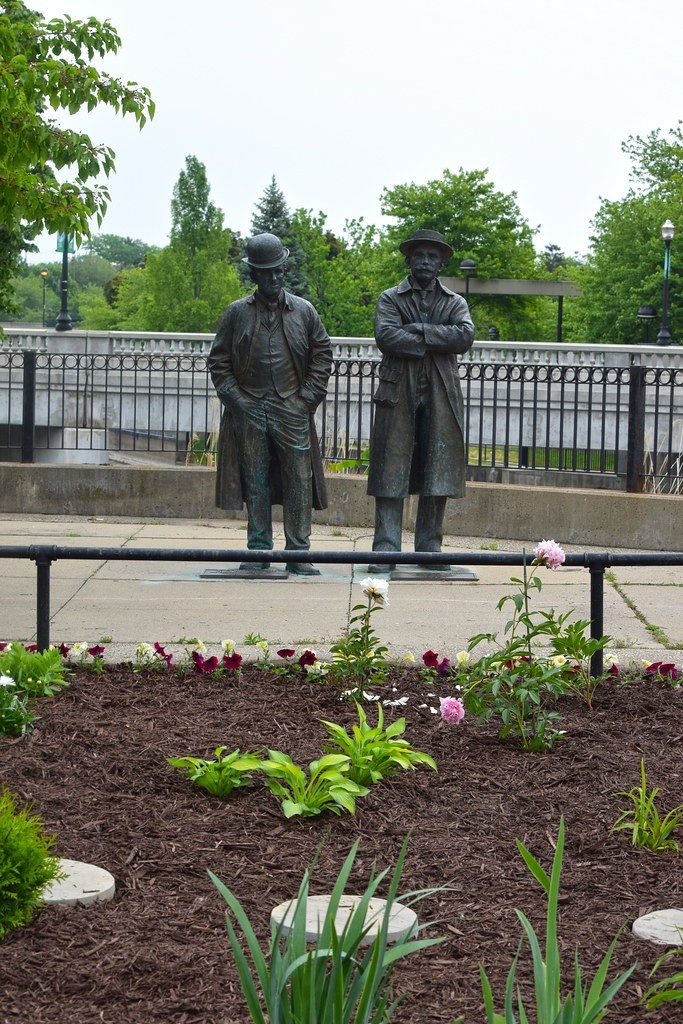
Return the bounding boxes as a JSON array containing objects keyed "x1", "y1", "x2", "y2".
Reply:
[
  {"x1": 656, "y1": 219, "x2": 675, "y2": 346},
  {"x1": 40, "y1": 268, "x2": 48, "y2": 327}
]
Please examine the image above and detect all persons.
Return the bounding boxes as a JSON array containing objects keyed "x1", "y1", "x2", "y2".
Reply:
[
  {"x1": 207, "y1": 233, "x2": 334, "y2": 575},
  {"x1": 363, "y1": 229, "x2": 476, "y2": 573}
]
